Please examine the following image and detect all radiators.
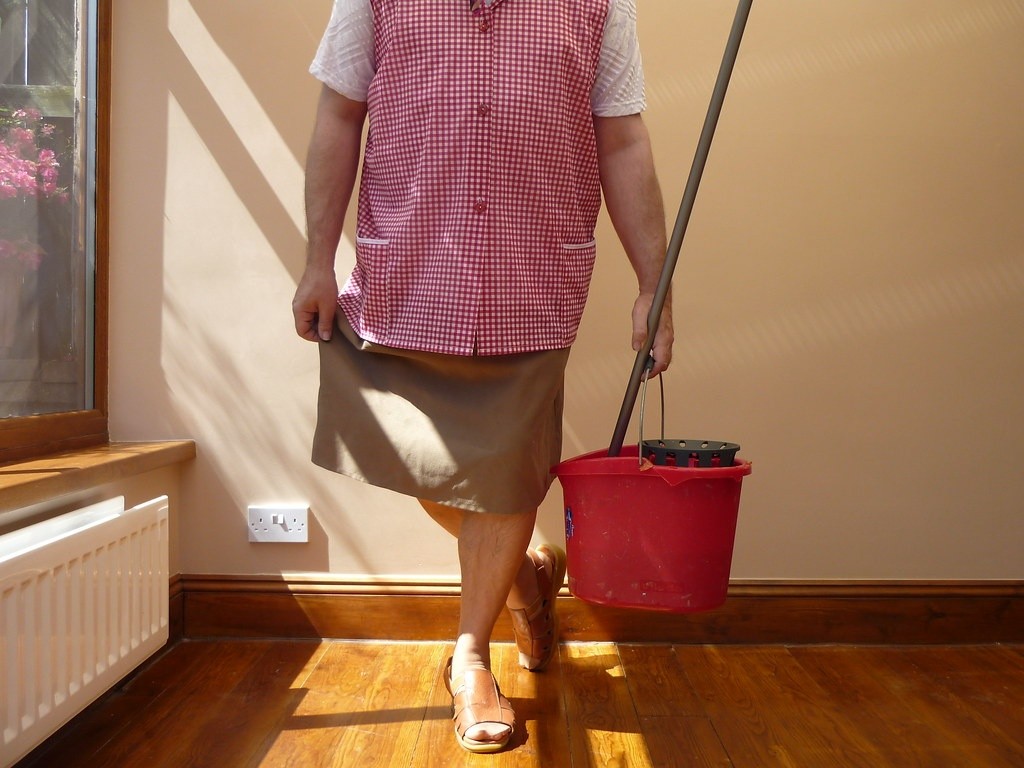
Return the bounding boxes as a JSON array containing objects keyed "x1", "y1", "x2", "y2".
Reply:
[{"x1": 0, "y1": 494, "x2": 172, "y2": 768}]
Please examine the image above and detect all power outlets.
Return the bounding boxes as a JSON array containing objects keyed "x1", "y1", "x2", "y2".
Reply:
[{"x1": 245, "y1": 504, "x2": 312, "y2": 545}]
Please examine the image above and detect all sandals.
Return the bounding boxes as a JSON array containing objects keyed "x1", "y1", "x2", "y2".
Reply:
[
  {"x1": 442, "y1": 655, "x2": 516, "y2": 752},
  {"x1": 505, "y1": 543, "x2": 567, "y2": 670}
]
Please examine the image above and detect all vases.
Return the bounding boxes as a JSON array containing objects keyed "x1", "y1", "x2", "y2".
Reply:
[{"x1": 0, "y1": 257, "x2": 23, "y2": 360}]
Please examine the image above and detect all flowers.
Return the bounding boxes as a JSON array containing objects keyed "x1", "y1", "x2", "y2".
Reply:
[{"x1": 0, "y1": 103, "x2": 72, "y2": 270}]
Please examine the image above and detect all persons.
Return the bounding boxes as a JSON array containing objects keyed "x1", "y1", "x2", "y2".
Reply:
[{"x1": 292, "y1": 0, "x2": 673, "y2": 755}]
